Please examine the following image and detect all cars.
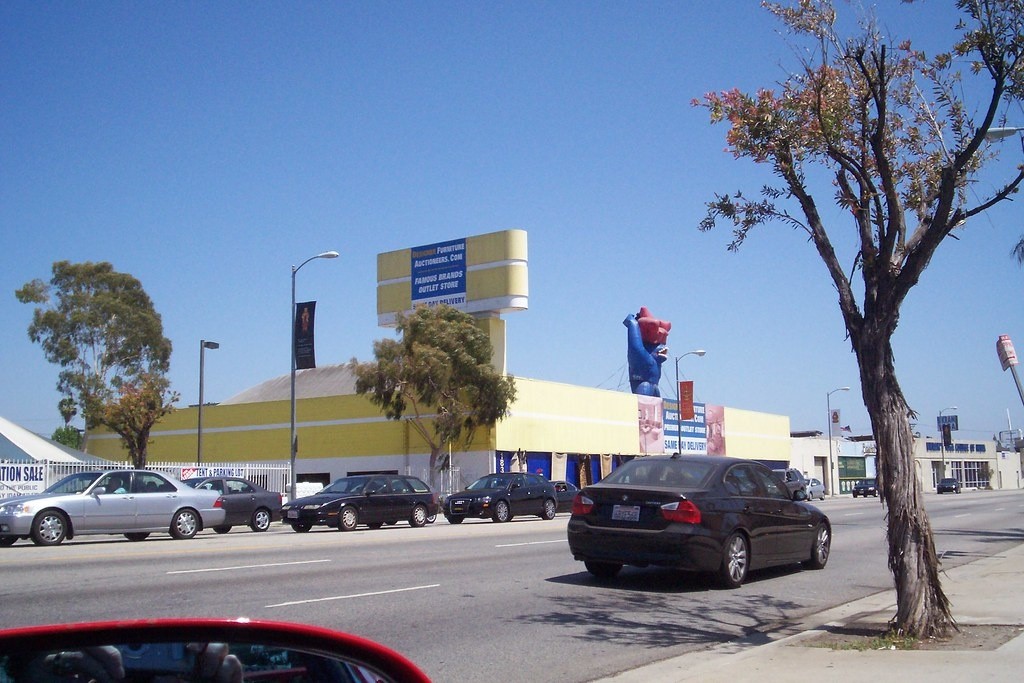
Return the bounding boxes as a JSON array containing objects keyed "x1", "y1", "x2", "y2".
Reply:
[
  {"x1": 366, "y1": 479, "x2": 440, "y2": 526},
  {"x1": 936, "y1": 478, "x2": 962, "y2": 494},
  {"x1": 0, "y1": 469, "x2": 227, "y2": 547},
  {"x1": 443, "y1": 472, "x2": 558, "y2": 524},
  {"x1": 852, "y1": 477, "x2": 878, "y2": 499},
  {"x1": 803, "y1": 477, "x2": 825, "y2": 501},
  {"x1": 549, "y1": 479, "x2": 583, "y2": 515},
  {"x1": 565, "y1": 455, "x2": 833, "y2": 588},
  {"x1": 149, "y1": 475, "x2": 282, "y2": 535}
]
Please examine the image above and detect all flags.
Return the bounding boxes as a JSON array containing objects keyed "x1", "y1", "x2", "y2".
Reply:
[{"x1": 841, "y1": 426, "x2": 851, "y2": 432}]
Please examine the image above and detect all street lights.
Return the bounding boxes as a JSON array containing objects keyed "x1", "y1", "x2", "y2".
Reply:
[
  {"x1": 196, "y1": 339, "x2": 220, "y2": 464},
  {"x1": 674, "y1": 350, "x2": 707, "y2": 456},
  {"x1": 826, "y1": 387, "x2": 850, "y2": 496},
  {"x1": 939, "y1": 406, "x2": 958, "y2": 478},
  {"x1": 288, "y1": 250, "x2": 340, "y2": 502}
]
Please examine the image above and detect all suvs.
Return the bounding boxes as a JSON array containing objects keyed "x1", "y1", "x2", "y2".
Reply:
[
  {"x1": 281, "y1": 473, "x2": 438, "y2": 534},
  {"x1": 761, "y1": 466, "x2": 807, "y2": 501}
]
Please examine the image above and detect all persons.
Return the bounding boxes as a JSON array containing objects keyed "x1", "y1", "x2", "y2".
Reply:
[
  {"x1": 211, "y1": 483, "x2": 223, "y2": 495},
  {"x1": 108, "y1": 478, "x2": 126, "y2": 494},
  {"x1": 92, "y1": 641, "x2": 244, "y2": 683}
]
